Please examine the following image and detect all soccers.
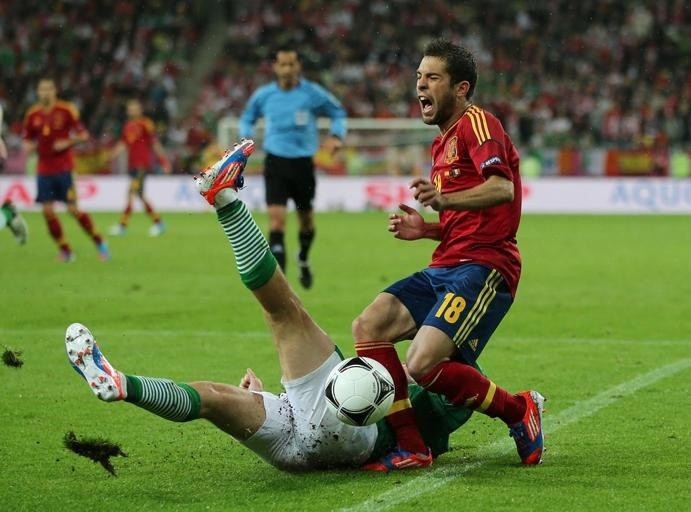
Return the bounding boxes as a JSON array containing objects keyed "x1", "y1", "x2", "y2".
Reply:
[{"x1": 325, "y1": 355, "x2": 396, "y2": 428}]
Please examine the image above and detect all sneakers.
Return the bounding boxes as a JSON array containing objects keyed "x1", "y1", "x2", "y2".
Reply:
[
  {"x1": 110, "y1": 225, "x2": 126, "y2": 236},
  {"x1": 299, "y1": 264, "x2": 311, "y2": 287},
  {"x1": 150, "y1": 224, "x2": 165, "y2": 237},
  {"x1": 193, "y1": 137, "x2": 257, "y2": 205},
  {"x1": 508, "y1": 390, "x2": 547, "y2": 466},
  {"x1": 64, "y1": 322, "x2": 122, "y2": 402},
  {"x1": 96, "y1": 244, "x2": 109, "y2": 262},
  {"x1": 60, "y1": 253, "x2": 74, "y2": 263},
  {"x1": 360, "y1": 446, "x2": 432, "y2": 473},
  {"x1": 2, "y1": 199, "x2": 27, "y2": 243}
]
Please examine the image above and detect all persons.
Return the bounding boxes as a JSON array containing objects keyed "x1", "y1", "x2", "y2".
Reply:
[
  {"x1": 65, "y1": 138, "x2": 487, "y2": 472},
  {"x1": 238, "y1": 45, "x2": 348, "y2": 290},
  {"x1": 352, "y1": 40, "x2": 546, "y2": 470},
  {"x1": 18, "y1": 80, "x2": 109, "y2": 261},
  {"x1": 111, "y1": 101, "x2": 169, "y2": 236},
  {"x1": 0, "y1": 1, "x2": 691, "y2": 173},
  {"x1": 1, "y1": 138, "x2": 29, "y2": 246}
]
